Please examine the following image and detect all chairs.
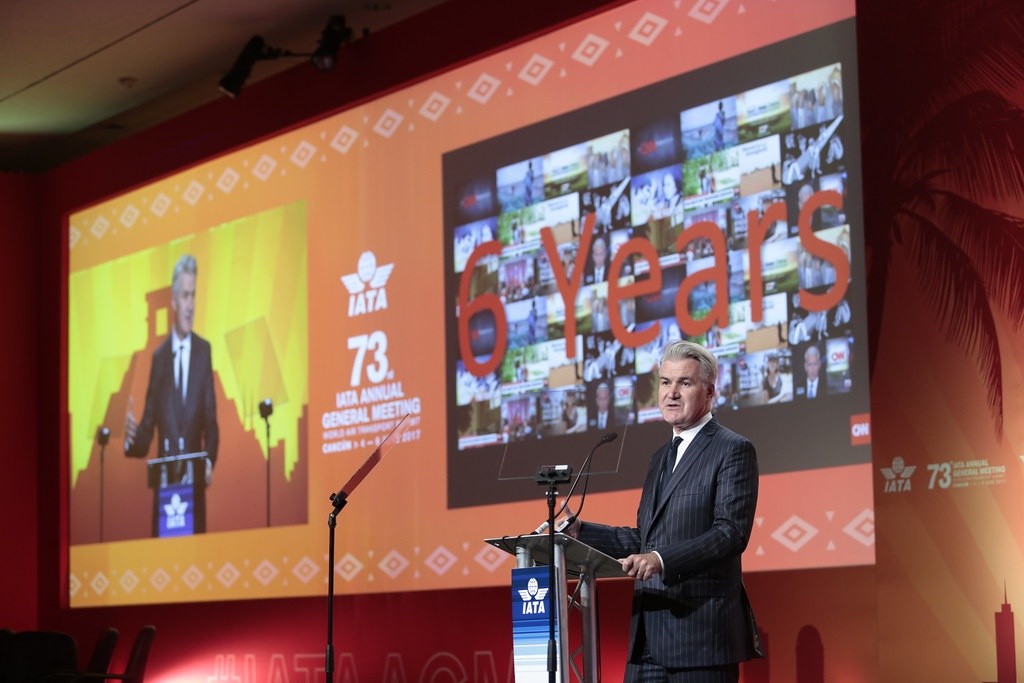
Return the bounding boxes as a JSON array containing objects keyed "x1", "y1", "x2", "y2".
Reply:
[{"x1": 0, "y1": 623, "x2": 156, "y2": 683}]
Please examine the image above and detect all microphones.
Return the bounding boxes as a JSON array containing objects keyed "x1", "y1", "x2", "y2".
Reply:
[{"x1": 530, "y1": 432, "x2": 618, "y2": 536}]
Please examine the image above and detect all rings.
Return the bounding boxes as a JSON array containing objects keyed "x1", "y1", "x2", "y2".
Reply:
[{"x1": 638, "y1": 571, "x2": 643, "y2": 575}]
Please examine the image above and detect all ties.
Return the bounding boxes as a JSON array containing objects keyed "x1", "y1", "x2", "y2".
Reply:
[
  {"x1": 597, "y1": 268, "x2": 602, "y2": 283},
  {"x1": 654, "y1": 437, "x2": 683, "y2": 509},
  {"x1": 809, "y1": 383, "x2": 813, "y2": 398},
  {"x1": 178, "y1": 346, "x2": 183, "y2": 403},
  {"x1": 600, "y1": 413, "x2": 604, "y2": 428}
]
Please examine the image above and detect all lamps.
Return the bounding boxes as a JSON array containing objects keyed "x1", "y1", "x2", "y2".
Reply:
[
  {"x1": 311, "y1": 14, "x2": 354, "y2": 70},
  {"x1": 218, "y1": 34, "x2": 314, "y2": 98}
]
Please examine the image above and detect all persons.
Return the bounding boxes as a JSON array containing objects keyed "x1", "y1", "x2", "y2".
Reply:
[
  {"x1": 555, "y1": 340, "x2": 759, "y2": 683},
  {"x1": 124, "y1": 253, "x2": 219, "y2": 486},
  {"x1": 456, "y1": 68, "x2": 848, "y2": 443}
]
